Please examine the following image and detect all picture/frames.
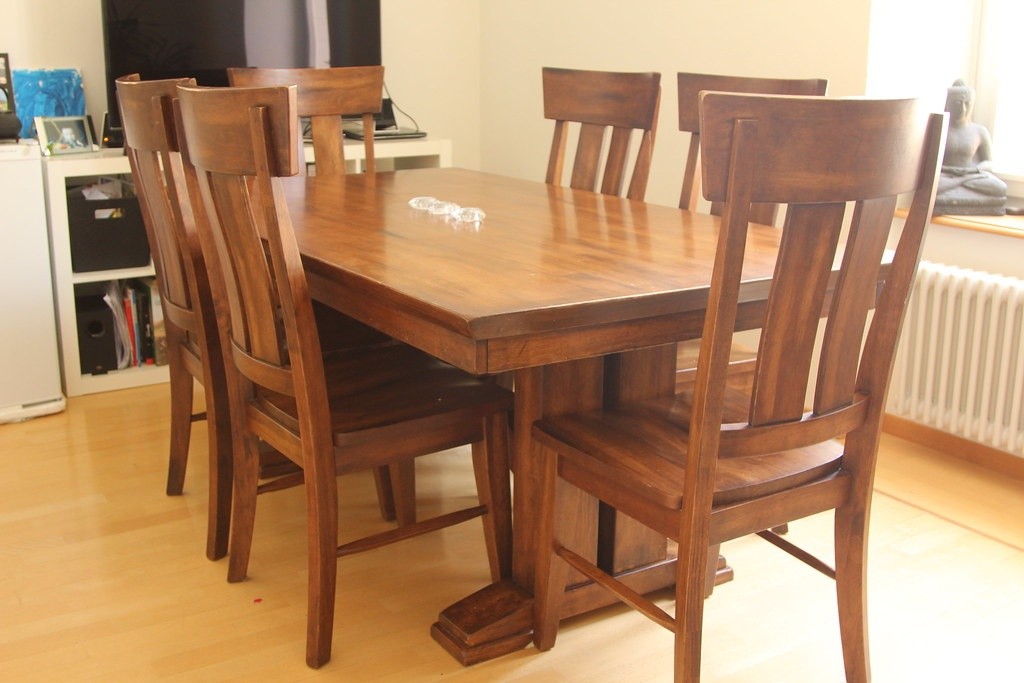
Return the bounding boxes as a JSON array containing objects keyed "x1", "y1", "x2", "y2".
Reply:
[{"x1": 42, "y1": 116, "x2": 94, "y2": 155}]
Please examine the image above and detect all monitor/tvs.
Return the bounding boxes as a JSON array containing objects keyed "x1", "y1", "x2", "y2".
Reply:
[{"x1": 101, "y1": 0, "x2": 381, "y2": 130}]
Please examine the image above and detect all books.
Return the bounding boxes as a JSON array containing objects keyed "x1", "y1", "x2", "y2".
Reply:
[{"x1": 101, "y1": 278, "x2": 169, "y2": 369}]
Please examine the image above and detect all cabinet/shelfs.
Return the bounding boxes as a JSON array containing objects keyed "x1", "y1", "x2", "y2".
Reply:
[{"x1": 42, "y1": 137, "x2": 452, "y2": 397}]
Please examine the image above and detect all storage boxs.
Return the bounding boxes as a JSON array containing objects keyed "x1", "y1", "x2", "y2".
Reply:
[{"x1": 67, "y1": 181, "x2": 149, "y2": 272}]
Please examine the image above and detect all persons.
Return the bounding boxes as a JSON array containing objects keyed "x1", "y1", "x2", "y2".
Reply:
[
  {"x1": 934, "y1": 78, "x2": 1007, "y2": 197},
  {"x1": 60, "y1": 126, "x2": 85, "y2": 148}
]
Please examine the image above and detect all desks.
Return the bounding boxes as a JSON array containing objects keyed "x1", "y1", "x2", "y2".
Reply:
[{"x1": 251, "y1": 165, "x2": 891, "y2": 668}]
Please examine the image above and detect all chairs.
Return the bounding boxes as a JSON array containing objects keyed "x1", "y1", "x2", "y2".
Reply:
[
  {"x1": 227, "y1": 65, "x2": 384, "y2": 175},
  {"x1": 542, "y1": 67, "x2": 663, "y2": 202},
  {"x1": 115, "y1": 74, "x2": 516, "y2": 670},
  {"x1": 529, "y1": 90, "x2": 949, "y2": 683},
  {"x1": 676, "y1": 73, "x2": 827, "y2": 423}
]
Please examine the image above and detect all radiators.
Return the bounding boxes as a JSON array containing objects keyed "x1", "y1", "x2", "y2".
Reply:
[{"x1": 858, "y1": 253, "x2": 1024, "y2": 462}]
